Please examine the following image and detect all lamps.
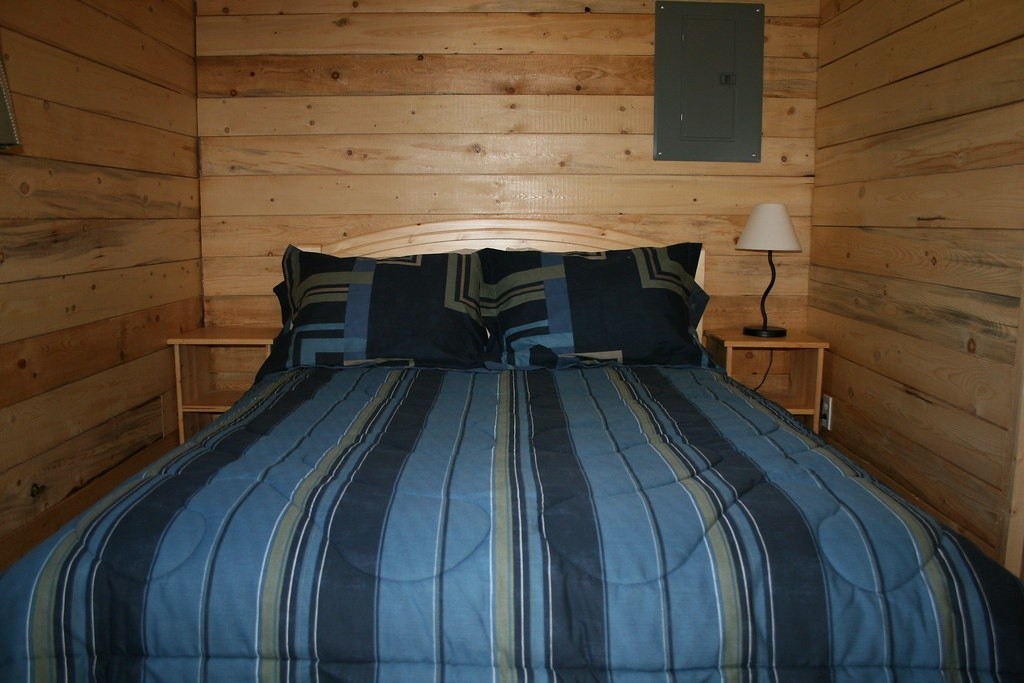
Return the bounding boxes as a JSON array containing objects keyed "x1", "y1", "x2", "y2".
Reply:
[{"x1": 735, "y1": 202, "x2": 802, "y2": 338}]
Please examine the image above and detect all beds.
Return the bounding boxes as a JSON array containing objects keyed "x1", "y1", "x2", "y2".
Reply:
[{"x1": 0, "y1": 216, "x2": 1024, "y2": 683}]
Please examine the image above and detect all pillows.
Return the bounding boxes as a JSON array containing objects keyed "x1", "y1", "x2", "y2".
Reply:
[{"x1": 254, "y1": 242, "x2": 723, "y2": 373}]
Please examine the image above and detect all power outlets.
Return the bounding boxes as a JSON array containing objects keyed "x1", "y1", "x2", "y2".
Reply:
[{"x1": 820, "y1": 395, "x2": 832, "y2": 430}]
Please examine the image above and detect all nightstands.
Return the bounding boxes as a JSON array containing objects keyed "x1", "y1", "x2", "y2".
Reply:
[
  {"x1": 703, "y1": 328, "x2": 830, "y2": 437},
  {"x1": 167, "y1": 325, "x2": 280, "y2": 446}
]
